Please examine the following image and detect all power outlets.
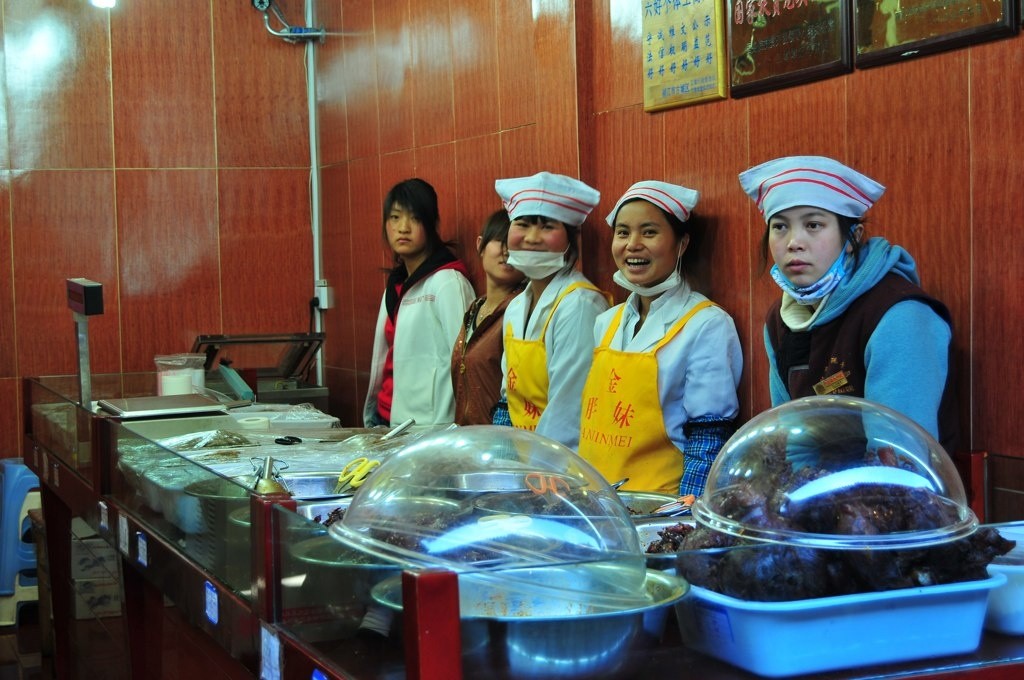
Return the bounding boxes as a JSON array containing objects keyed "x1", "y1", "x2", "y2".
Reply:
[{"x1": 315, "y1": 287, "x2": 328, "y2": 309}]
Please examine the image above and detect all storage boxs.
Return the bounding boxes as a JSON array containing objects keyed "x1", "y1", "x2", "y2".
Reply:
[
  {"x1": 72, "y1": 578, "x2": 122, "y2": 620},
  {"x1": 71, "y1": 517, "x2": 97, "y2": 540},
  {"x1": 71, "y1": 536, "x2": 120, "y2": 579}
]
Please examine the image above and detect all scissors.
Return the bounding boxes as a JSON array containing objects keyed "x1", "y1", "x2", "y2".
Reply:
[
  {"x1": 525, "y1": 473, "x2": 572, "y2": 505},
  {"x1": 654, "y1": 494, "x2": 696, "y2": 512},
  {"x1": 332, "y1": 457, "x2": 381, "y2": 494},
  {"x1": 275, "y1": 437, "x2": 303, "y2": 446}
]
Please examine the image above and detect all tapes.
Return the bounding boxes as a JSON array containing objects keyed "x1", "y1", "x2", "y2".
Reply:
[{"x1": 238, "y1": 417, "x2": 270, "y2": 430}]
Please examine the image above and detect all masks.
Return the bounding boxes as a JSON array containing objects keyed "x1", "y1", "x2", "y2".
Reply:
[
  {"x1": 613, "y1": 270, "x2": 682, "y2": 295},
  {"x1": 768, "y1": 258, "x2": 845, "y2": 307},
  {"x1": 507, "y1": 249, "x2": 568, "y2": 281}
]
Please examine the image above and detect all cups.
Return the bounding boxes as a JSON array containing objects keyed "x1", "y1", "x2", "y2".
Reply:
[{"x1": 162, "y1": 376, "x2": 191, "y2": 395}]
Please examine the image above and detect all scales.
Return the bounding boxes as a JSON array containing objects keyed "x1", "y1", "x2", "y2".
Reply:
[{"x1": 66, "y1": 276, "x2": 243, "y2": 440}]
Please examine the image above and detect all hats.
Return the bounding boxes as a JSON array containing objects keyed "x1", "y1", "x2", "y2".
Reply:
[
  {"x1": 605, "y1": 180, "x2": 699, "y2": 227},
  {"x1": 737, "y1": 156, "x2": 887, "y2": 224},
  {"x1": 495, "y1": 172, "x2": 601, "y2": 226}
]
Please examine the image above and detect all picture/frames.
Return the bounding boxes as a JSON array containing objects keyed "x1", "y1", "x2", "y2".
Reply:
[
  {"x1": 852, "y1": 0, "x2": 1020, "y2": 70},
  {"x1": 726, "y1": 0, "x2": 855, "y2": 100}
]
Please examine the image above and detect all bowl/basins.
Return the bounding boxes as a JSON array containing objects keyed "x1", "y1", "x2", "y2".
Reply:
[{"x1": 182, "y1": 472, "x2": 1024, "y2": 680}]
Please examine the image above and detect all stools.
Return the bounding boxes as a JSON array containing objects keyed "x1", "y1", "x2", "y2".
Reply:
[
  {"x1": 0, "y1": 456, "x2": 40, "y2": 597},
  {"x1": 0, "y1": 491, "x2": 42, "y2": 627},
  {"x1": 0, "y1": 516, "x2": 38, "y2": 631}
]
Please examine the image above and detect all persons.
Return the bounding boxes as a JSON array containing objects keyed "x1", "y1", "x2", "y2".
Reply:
[
  {"x1": 363, "y1": 178, "x2": 476, "y2": 424},
  {"x1": 494, "y1": 170, "x2": 613, "y2": 472},
  {"x1": 450, "y1": 208, "x2": 528, "y2": 430},
  {"x1": 739, "y1": 156, "x2": 973, "y2": 512},
  {"x1": 578, "y1": 180, "x2": 743, "y2": 500}
]
